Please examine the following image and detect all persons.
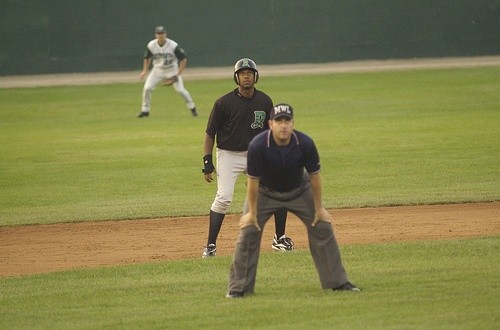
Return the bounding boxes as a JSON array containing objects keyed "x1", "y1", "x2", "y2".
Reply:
[
  {"x1": 224, "y1": 104, "x2": 363, "y2": 299},
  {"x1": 202, "y1": 58, "x2": 295, "y2": 258},
  {"x1": 137, "y1": 26, "x2": 198, "y2": 118}
]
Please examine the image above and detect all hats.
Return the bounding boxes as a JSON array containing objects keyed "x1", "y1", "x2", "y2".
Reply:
[
  {"x1": 155, "y1": 25, "x2": 166, "y2": 34},
  {"x1": 270, "y1": 103, "x2": 293, "y2": 120}
]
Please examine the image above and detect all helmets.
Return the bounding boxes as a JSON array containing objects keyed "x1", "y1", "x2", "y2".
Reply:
[{"x1": 233, "y1": 58, "x2": 259, "y2": 85}]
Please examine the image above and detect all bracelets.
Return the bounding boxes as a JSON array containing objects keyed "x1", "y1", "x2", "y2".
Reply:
[{"x1": 172, "y1": 76, "x2": 178, "y2": 81}]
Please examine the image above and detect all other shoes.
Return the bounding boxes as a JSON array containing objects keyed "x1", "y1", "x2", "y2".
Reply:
[
  {"x1": 226, "y1": 291, "x2": 244, "y2": 298},
  {"x1": 138, "y1": 111, "x2": 149, "y2": 117},
  {"x1": 332, "y1": 281, "x2": 360, "y2": 292},
  {"x1": 190, "y1": 107, "x2": 198, "y2": 117}
]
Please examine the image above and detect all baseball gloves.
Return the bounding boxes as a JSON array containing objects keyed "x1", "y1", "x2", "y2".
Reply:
[{"x1": 163, "y1": 75, "x2": 178, "y2": 86}]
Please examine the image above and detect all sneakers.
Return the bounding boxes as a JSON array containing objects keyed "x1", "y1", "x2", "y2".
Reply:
[
  {"x1": 202, "y1": 243, "x2": 217, "y2": 259},
  {"x1": 271, "y1": 234, "x2": 294, "y2": 252}
]
